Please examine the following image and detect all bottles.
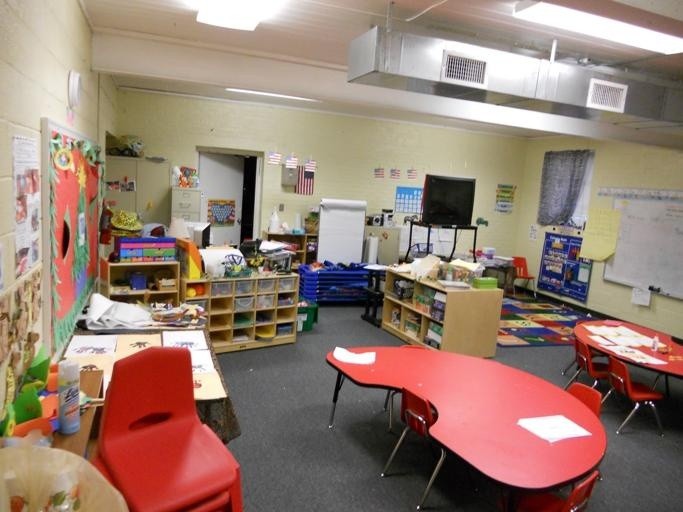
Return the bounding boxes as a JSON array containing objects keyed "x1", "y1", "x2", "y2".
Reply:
[
  {"x1": 650, "y1": 332, "x2": 659, "y2": 352},
  {"x1": 56, "y1": 359, "x2": 81, "y2": 435},
  {"x1": 268, "y1": 208, "x2": 279, "y2": 234}
]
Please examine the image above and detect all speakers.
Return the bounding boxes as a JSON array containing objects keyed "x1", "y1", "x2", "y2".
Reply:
[
  {"x1": 368, "y1": 214, "x2": 383, "y2": 226},
  {"x1": 383, "y1": 213, "x2": 394, "y2": 226}
]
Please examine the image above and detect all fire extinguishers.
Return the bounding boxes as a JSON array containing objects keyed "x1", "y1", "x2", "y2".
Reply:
[{"x1": 100, "y1": 207, "x2": 113, "y2": 244}]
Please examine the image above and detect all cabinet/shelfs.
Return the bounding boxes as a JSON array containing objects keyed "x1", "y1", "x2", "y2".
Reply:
[
  {"x1": 381, "y1": 264, "x2": 504, "y2": 358},
  {"x1": 262, "y1": 229, "x2": 318, "y2": 274},
  {"x1": 171, "y1": 185, "x2": 202, "y2": 222},
  {"x1": 365, "y1": 225, "x2": 399, "y2": 267},
  {"x1": 180, "y1": 271, "x2": 300, "y2": 356},
  {"x1": 100, "y1": 255, "x2": 181, "y2": 308}
]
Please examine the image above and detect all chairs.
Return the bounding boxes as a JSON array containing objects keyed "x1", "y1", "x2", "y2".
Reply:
[
  {"x1": 510, "y1": 256, "x2": 538, "y2": 298},
  {"x1": 563, "y1": 319, "x2": 666, "y2": 438},
  {"x1": 380, "y1": 385, "x2": 445, "y2": 511},
  {"x1": 327, "y1": 344, "x2": 438, "y2": 432},
  {"x1": 517, "y1": 470, "x2": 600, "y2": 512},
  {"x1": 90, "y1": 344, "x2": 243, "y2": 512}
]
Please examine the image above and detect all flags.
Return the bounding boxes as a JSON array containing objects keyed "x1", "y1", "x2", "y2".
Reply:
[
  {"x1": 373, "y1": 168, "x2": 384, "y2": 179},
  {"x1": 388, "y1": 168, "x2": 400, "y2": 179},
  {"x1": 294, "y1": 165, "x2": 314, "y2": 195},
  {"x1": 406, "y1": 169, "x2": 417, "y2": 180},
  {"x1": 284, "y1": 153, "x2": 297, "y2": 168},
  {"x1": 265, "y1": 151, "x2": 281, "y2": 165},
  {"x1": 303, "y1": 158, "x2": 316, "y2": 172}
]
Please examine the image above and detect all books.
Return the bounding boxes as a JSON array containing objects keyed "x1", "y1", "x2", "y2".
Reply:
[{"x1": 437, "y1": 278, "x2": 470, "y2": 290}]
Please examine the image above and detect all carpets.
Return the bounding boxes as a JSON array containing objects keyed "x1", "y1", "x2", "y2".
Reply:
[{"x1": 496, "y1": 286, "x2": 604, "y2": 348}]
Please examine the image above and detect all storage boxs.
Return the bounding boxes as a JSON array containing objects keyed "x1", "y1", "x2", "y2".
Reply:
[
  {"x1": 277, "y1": 325, "x2": 293, "y2": 337},
  {"x1": 211, "y1": 278, "x2": 296, "y2": 311}
]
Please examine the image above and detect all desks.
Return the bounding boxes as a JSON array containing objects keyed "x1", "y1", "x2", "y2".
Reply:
[
  {"x1": 325, "y1": 346, "x2": 607, "y2": 512},
  {"x1": 482, "y1": 264, "x2": 516, "y2": 294},
  {"x1": 55, "y1": 324, "x2": 242, "y2": 445},
  {"x1": 0, "y1": 369, "x2": 105, "y2": 464}
]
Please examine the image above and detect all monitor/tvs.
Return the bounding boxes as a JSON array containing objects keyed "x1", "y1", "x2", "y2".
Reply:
[{"x1": 422, "y1": 175, "x2": 476, "y2": 225}]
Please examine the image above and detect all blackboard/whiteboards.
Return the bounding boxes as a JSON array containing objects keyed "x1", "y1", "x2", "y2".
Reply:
[{"x1": 603, "y1": 194, "x2": 682, "y2": 301}]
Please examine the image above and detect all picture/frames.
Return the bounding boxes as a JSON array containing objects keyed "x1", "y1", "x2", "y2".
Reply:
[{"x1": 40, "y1": 115, "x2": 101, "y2": 368}]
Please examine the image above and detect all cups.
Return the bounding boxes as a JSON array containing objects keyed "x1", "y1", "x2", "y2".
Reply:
[{"x1": 481, "y1": 247, "x2": 496, "y2": 262}]
